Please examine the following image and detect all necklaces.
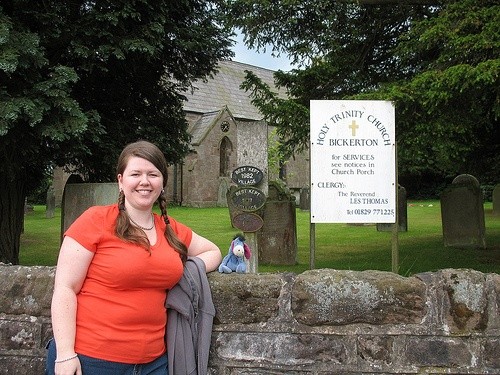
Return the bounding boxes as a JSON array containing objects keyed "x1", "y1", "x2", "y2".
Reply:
[{"x1": 124, "y1": 211, "x2": 154, "y2": 230}]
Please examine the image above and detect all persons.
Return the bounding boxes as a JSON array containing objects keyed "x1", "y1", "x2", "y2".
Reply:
[{"x1": 44, "y1": 141, "x2": 222, "y2": 375}]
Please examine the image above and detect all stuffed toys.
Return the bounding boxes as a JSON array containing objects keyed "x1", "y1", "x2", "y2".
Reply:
[{"x1": 218, "y1": 233, "x2": 251, "y2": 273}]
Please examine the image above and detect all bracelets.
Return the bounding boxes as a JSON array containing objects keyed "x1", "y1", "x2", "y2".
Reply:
[{"x1": 54, "y1": 353, "x2": 78, "y2": 363}]
